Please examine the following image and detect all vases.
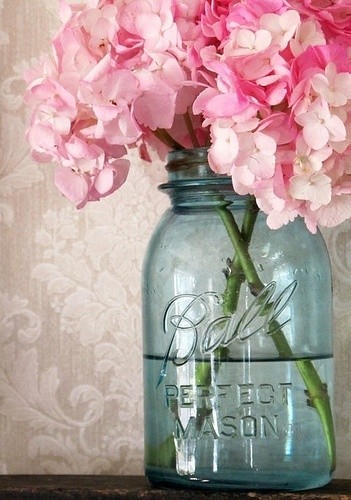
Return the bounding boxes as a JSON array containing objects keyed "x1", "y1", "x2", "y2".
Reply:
[{"x1": 139, "y1": 145, "x2": 335, "y2": 490}]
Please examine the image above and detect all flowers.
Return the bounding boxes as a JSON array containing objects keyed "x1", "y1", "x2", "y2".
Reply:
[{"x1": 22, "y1": 0, "x2": 351, "y2": 469}]
